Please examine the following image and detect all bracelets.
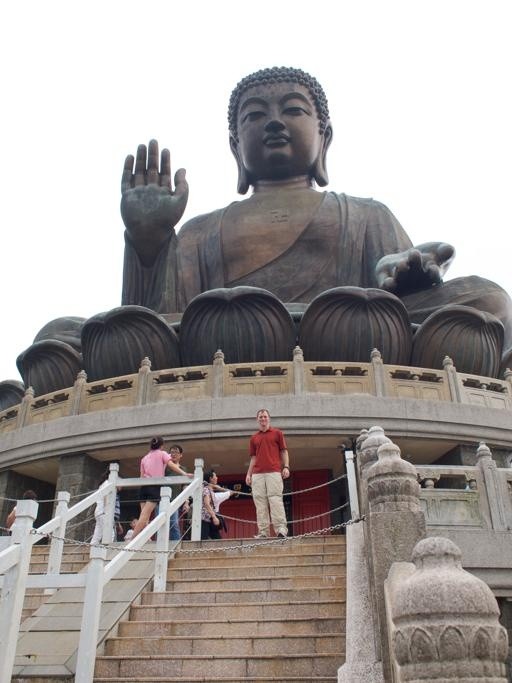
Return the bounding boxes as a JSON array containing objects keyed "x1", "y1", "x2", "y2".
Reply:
[{"x1": 283, "y1": 466, "x2": 289, "y2": 469}]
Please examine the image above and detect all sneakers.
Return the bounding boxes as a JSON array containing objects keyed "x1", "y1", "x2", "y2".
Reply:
[
  {"x1": 277, "y1": 531, "x2": 287, "y2": 537},
  {"x1": 254, "y1": 533, "x2": 266, "y2": 538}
]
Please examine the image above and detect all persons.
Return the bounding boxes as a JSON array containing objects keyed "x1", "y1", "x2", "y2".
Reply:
[
  {"x1": 245, "y1": 408, "x2": 291, "y2": 538},
  {"x1": 90, "y1": 471, "x2": 116, "y2": 545},
  {"x1": 28, "y1": 66, "x2": 512, "y2": 357},
  {"x1": 5, "y1": 488, "x2": 37, "y2": 536},
  {"x1": 128, "y1": 435, "x2": 195, "y2": 538},
  {"x1": 113, "y1": 469, "x2": 240, "y2": 541},
  {"x1": 153, "y1": 442, "x2": 182, "y2": 539}
]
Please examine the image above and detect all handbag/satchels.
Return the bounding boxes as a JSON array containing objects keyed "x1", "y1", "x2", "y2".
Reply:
[{"x1": 211, "y1": 515, "x2": 224, "y2": 530}]
